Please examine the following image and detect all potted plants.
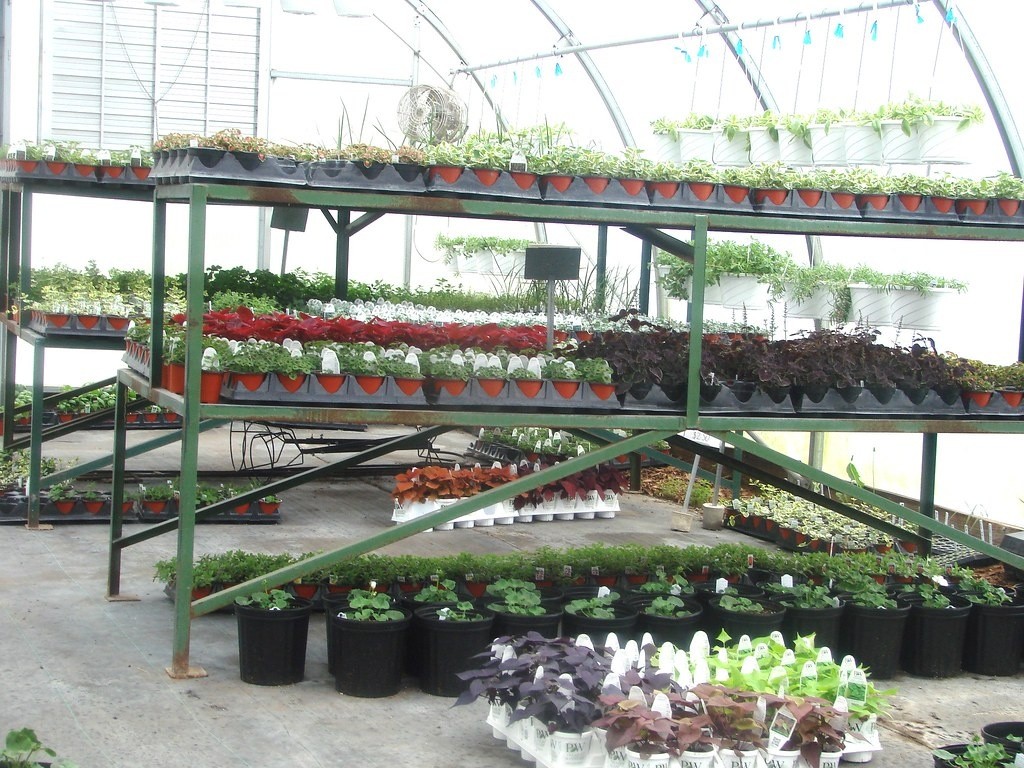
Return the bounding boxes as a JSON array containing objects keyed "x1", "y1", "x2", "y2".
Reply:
[{"x1": 0, "y1": 105, "x2": 1024, "y2": 768}]
